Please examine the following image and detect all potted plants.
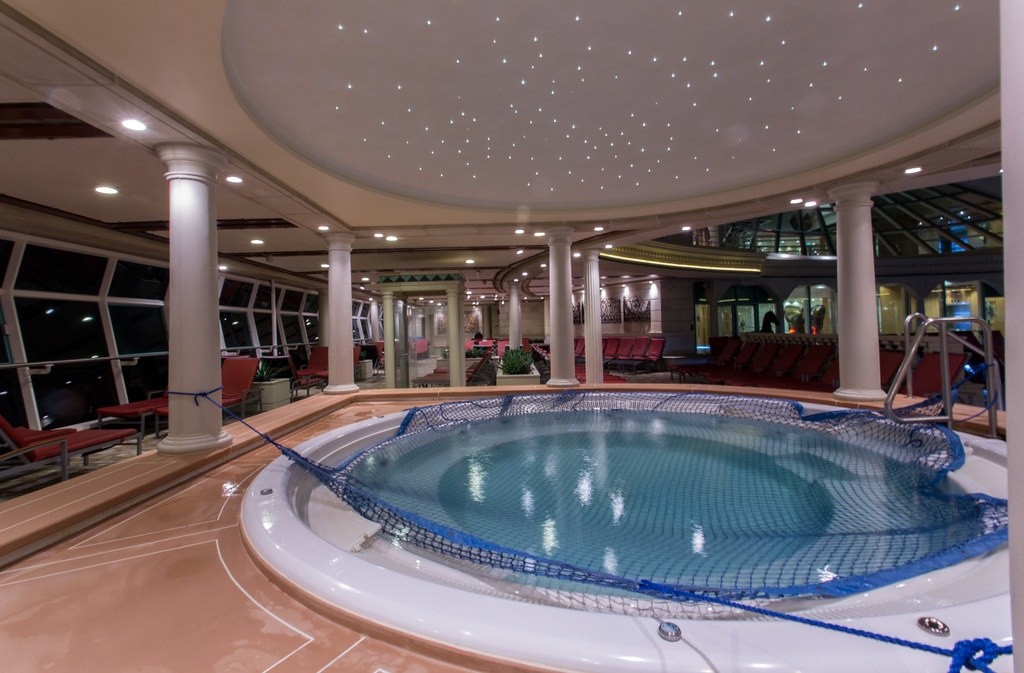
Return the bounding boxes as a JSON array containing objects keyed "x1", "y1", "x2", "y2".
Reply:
[
  {"x1": 466, "y1": 348, "x2": 483, "y2": 368},
  {"x1": 240, "y1": 357, "x2": 290, "y2": 412},
  {"x1": 357, "y1": 351, "x2": 373, "y2": 379},
  {"x1": 494, "y1": 348, "x2": 541, "y2": 385}
]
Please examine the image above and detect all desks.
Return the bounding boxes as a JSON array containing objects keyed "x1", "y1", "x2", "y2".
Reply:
[{"x1": 663, "y1": 355, "x2": 706, "y2": 383}]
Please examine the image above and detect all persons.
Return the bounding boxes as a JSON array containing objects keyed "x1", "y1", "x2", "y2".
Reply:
[{"x1": 475, "y1": 333, "x2": 483, "y2": 340}]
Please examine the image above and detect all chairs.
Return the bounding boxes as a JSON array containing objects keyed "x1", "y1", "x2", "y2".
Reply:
[
  {"x1": 291, "y1": 334, "x2": 666, "y2": 397},
  {"x1": 672, "y1": 333, "x2": 967, "y2": 399},
  {"x1": 0, "y1": 350, "x2": 259, "y2": 467}
]
